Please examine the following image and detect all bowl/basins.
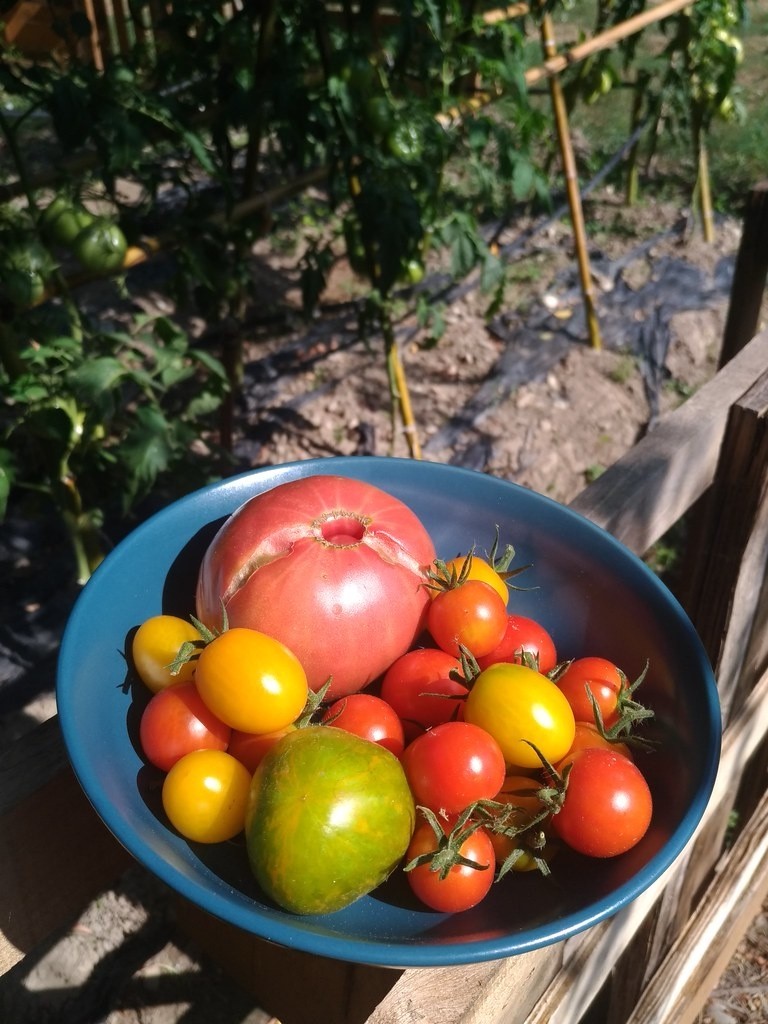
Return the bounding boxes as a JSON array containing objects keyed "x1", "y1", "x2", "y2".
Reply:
[{"x1": 55, "y1": 456, "x2": 723, "y2": 966}]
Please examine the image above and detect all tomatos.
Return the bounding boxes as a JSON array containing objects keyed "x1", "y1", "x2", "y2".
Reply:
[{"x1": 132, "y1": 474, "x2": 654, "y2": 917}]
